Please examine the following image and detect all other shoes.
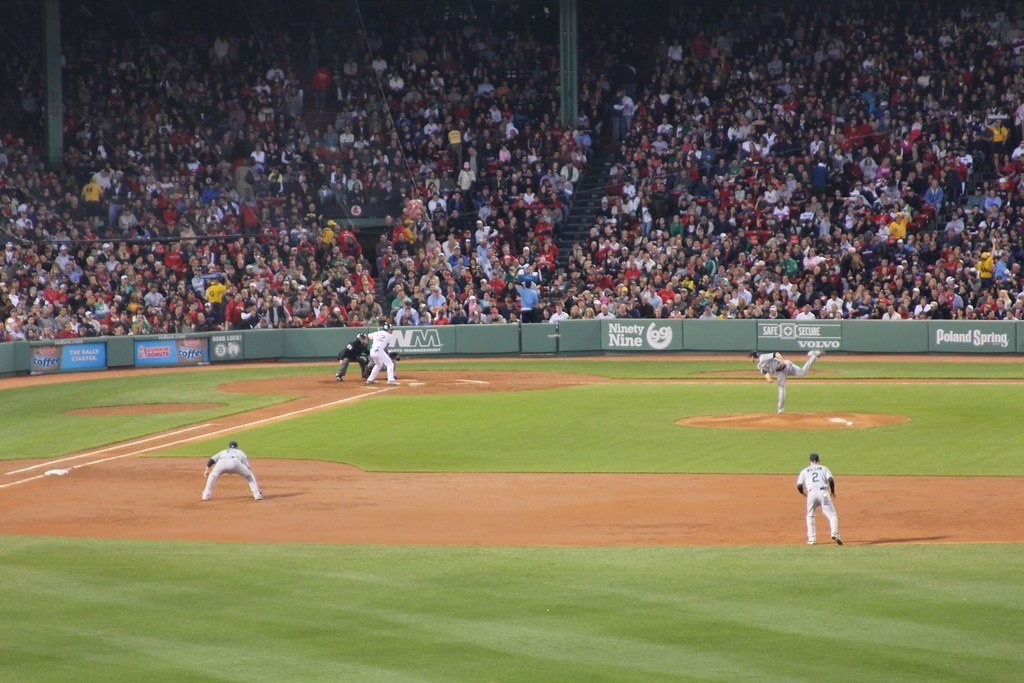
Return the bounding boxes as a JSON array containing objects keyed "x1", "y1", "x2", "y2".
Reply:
[
  {"x1": 814, "y1": 350, "x2": 825, "y2": 356},
  {"x1": 366, "y1": 380, "x2": 377, "y2": 384},
  {"x1": 808, "y1": 541, "x2": 815, "y2": 545},
  {"x1": 336, "y1": 374, "x2": 342, "y2": 381},
  {"x1": 832, "y1": 535, "x2": 843, "y2": 545},
  {"x1": 255, "y1": 496, "x2": 262, "y2": 500},
  {"x1": 201, "y1": 496, "x2": 208, "y2": 501},
  {"x1": 386, "y1": 381, "x2": 399, "y2": 386},
  {"x1": 777, "y1": 411, "x2": 783, "y2": 413}
]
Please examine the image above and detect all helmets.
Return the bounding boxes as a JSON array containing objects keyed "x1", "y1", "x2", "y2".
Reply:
[
  {"x1": 357, "y1": 333, "x2": 367, "y2": 341},
  {"x1": 383, "y1": 324, "x2": 393, "y2": 331}
]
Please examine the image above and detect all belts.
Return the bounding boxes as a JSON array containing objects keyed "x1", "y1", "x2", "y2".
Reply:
[{"x1": 809, "y1": 488, "x2": 825, "y2": 491}]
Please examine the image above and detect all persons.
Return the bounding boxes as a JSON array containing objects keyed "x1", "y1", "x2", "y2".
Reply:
[
  {"x1": 597, "y1": 304, "x2": 615, "y2": 319},
  {"x1": 336, "y1": 322, "x2": 400, "y2": 386},
  {"x1": 516, "y1": 280, "x2": 539, "y2": 323},
  {"x1": 748, "y1": 349, "x2": 823, "y2": 414},
  {"x1": 201, "y1": 441, "x2": 263, "y2": 500},
  {"x1": 550, "y1": 306, "x2": 569, "y2": 323},
  {"x1": 796, "y1": 304, "x2": 815, "y2": 320},
  {"x1": 0, "y1": 0, "x2": 1024, "y2": 343},
  {"x1": 796, "y1": 454, "x2": 843, "y2": 545}
]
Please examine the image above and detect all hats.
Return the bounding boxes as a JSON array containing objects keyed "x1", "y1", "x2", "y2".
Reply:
[
  {"x1": 230, "y1": 441, "x2": 237, "y2": 448},
  {"x1": 810, "y1": 453, "x2": 818, "y2": 461},
  {"x1": 747, "y1": 350, "x2": 757, "y2": 357}
]
